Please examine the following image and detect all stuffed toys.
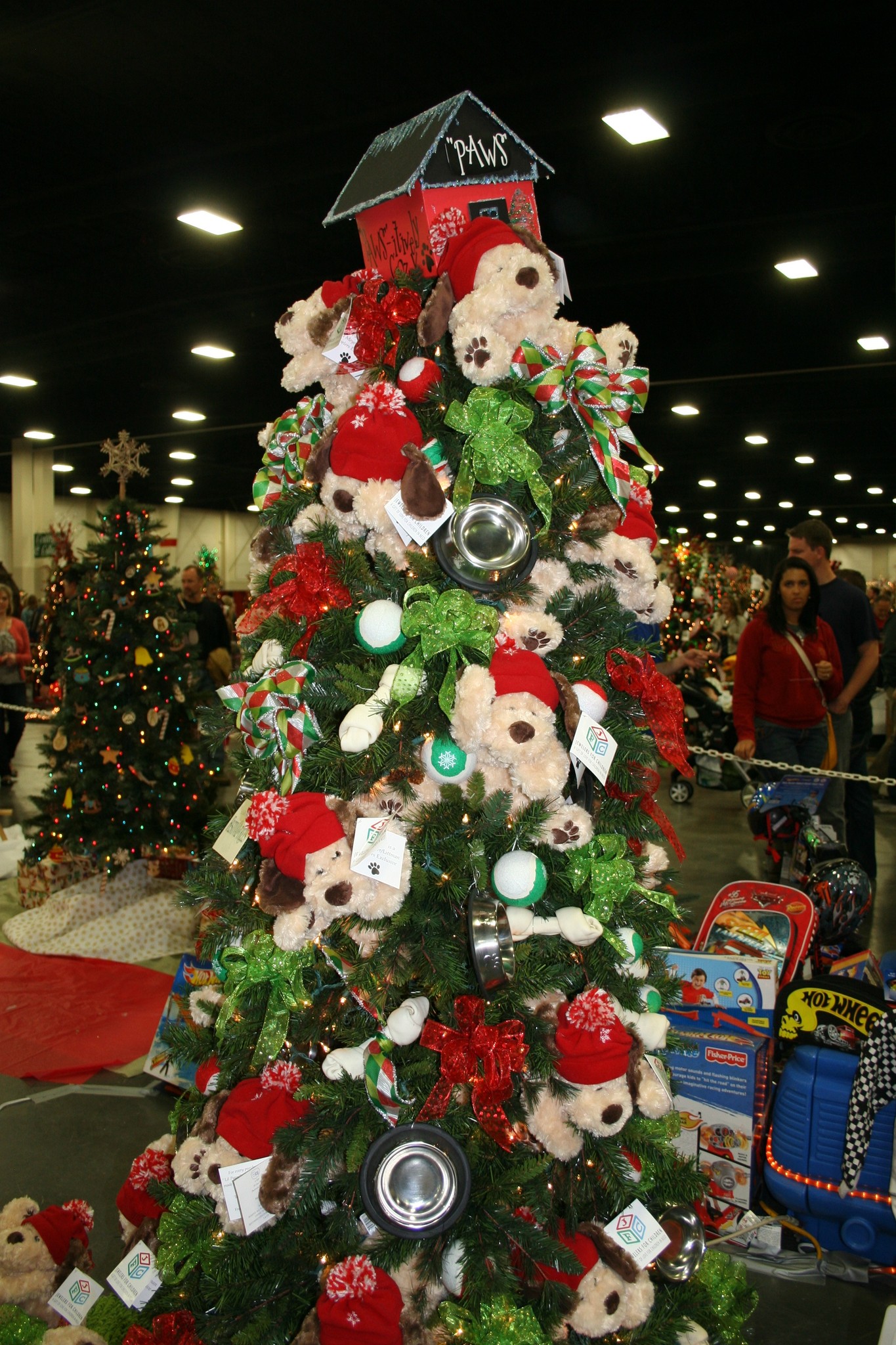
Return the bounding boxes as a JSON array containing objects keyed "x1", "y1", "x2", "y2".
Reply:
[
  {"x1": 513, "y1": 990, "x2": 670, "y2": 1159},
  {"x1": 510, "y1": 1205, "x2": 710, "y2": 1345},
  {"x1": 258, "y1": 217, "x2": 673, "y2": 654},
  {"x1": 245, "y1": 640, "x2": 669, "y2": 954},
  {"x1": 0, "y1": 1197, "x2": 108, "y2": 1345},
  {"x1": 116, "y1": 1060, "x2": 343, "y2": 1248},
  {"x1": 316, "y1": 1221, "x2": 495, "y2": 1345}
]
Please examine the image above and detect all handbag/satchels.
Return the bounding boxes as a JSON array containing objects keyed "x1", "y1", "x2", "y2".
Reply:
[{"x1": 820, "y1": 714, "x2": 838, "y2": 771}]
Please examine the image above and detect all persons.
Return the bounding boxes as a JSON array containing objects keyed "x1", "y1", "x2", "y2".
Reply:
[
  {"x1": 0, "y1": 584, "x2": 32, "y2": 786},
  {"x1": 175, "y1": 564, "x2": 233, "y2": 786},
  {"x1": 714, "y1": 595, "x2": 746, "y2": 655},
  {"x1": 629, "y1": 578, "x2": 720, "y2": 676},
  {"x1": 874, "y1": 596, "x2": 893, "y2": 682},
  {"x1": 23, "y1": 595, "x2": 37, "y2": 634},
  {"x1": 733, "y1": 557, "x2": 843, "y2": 884},
  {"x1": 787, "y1": 519, "x2": 881, "y2": 849},
  {"x1": 63, "y1": 568, "x2": 81, "y2": 599},
  {"x1": 672, "y1": 964, "x2": 727, "y2": 1009},
  {"x1": 207, "y1": 583, "x2": 225, "y2": 605},
  {"x1": 881, "y1": 611, "x2": 896, "y2": 804},
  {"x1": 838, "y1": 569, "x2": 877, "y2": 880}
]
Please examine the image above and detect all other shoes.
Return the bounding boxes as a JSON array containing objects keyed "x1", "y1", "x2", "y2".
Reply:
[{"x1": 1, "y1": 776, "x2": 12, "y2": 785}]
